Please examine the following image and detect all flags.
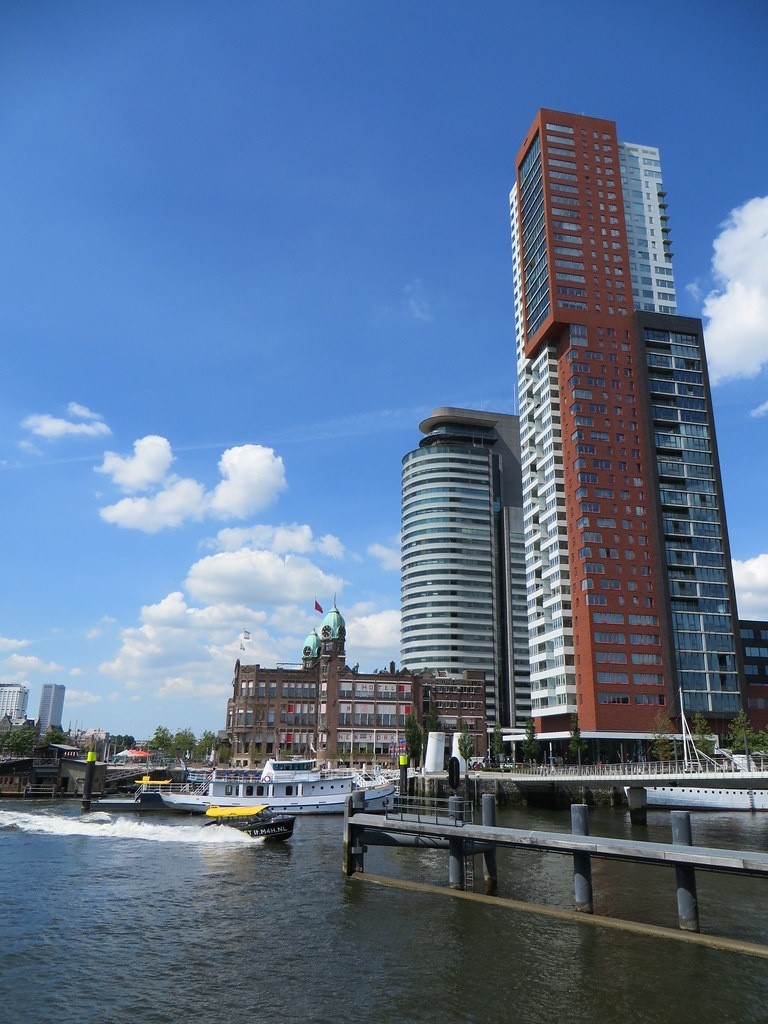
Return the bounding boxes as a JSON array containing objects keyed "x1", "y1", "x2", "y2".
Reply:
[
  {"x1": 314, "y1": 600, "x2": 323, "y2": 613},
  {"x1": 240, "y1": 630, "x2": 250, "y2": 650}
]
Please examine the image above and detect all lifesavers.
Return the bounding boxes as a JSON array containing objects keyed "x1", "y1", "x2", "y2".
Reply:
[
  {"x1": 206, "y1": 774, "x2": 213, "y2": 781},
  {"x1": 264, "y1": 776, "x2": 271, "y2": 783}
]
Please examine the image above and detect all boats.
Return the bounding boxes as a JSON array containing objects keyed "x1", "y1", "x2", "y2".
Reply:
[
  {"x1": 622, "y1": 687, "x2": 768, "y2": 812},
  {"x1": 206, "y1": 805, "x2": 297, "y2": 845},
  {"x1": 134, "y1": 757, "x2": 397, "y2": 816}
]
